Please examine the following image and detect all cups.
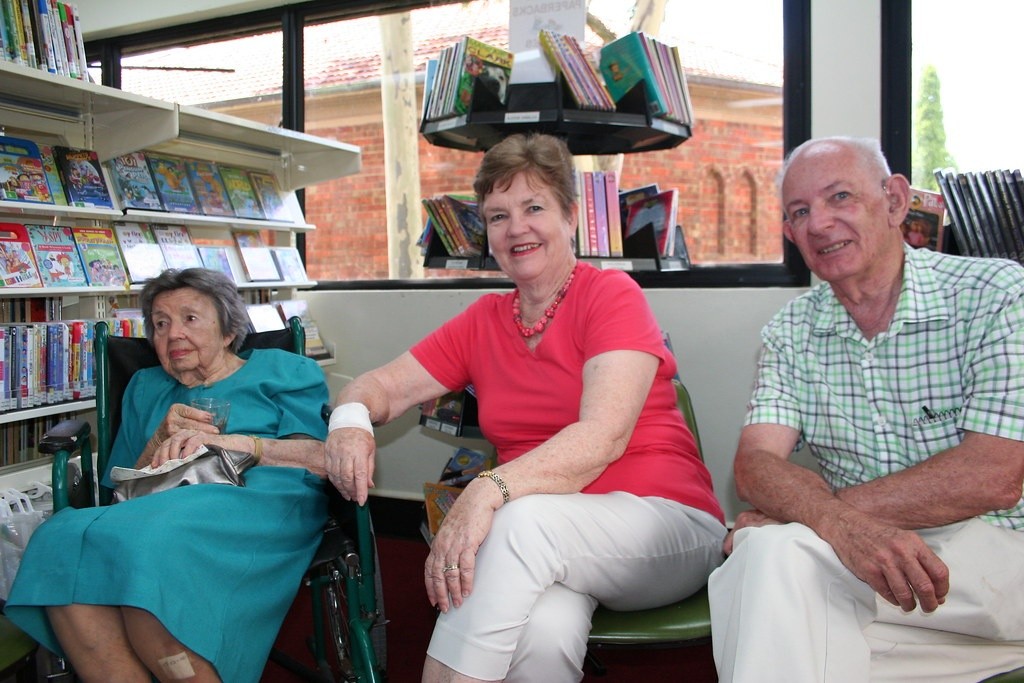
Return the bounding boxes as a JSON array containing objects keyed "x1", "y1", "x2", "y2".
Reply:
[{"x1": 191, "y1": 398, "x2": 231, "y2": 435}]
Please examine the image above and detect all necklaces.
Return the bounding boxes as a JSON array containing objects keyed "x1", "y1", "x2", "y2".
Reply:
[{"x1": 512, "y1": 267, "x2": 576, "y2": 338}]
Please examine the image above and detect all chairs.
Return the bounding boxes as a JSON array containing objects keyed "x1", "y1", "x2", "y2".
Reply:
[{"x1": 495, "y1": 379, "x2": 711, "y2": 675}]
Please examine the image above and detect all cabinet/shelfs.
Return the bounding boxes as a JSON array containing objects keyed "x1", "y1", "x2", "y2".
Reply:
[
  {"x1": 0, "y1": 56, "x2": 363, "y2": 508},
  {"x1": 421, "y1": 70, "x2": 694, "y2": 273}
]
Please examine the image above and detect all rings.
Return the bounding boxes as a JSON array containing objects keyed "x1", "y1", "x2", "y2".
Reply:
[{"x1": 443, "y1": 564, "x2": 460, "y2": 572}]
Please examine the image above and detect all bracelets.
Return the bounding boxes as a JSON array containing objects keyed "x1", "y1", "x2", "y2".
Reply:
[
  {"x1": 249, "y1": 435, "x2": 262, "y2": 464},
  {"x1": 328, "y1": 403, "x2": 374, "y2": 438},
  {"x1": 477, "y1": 470, "x2": 509, "y2": 504}
]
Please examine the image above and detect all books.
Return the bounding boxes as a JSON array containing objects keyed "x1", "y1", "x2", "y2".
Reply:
[
  {"x1": 899, "y1": 187, "x2": 945, "y2": 253},
  {"x1": 539, "y1": 29, "x2": 694, "y2": 127},
  {"x1": 0, "y1": 135, "x2": 331, "y2": 467},
  {"x1": 0, "y1": 0, "x2": 90, "y2": 82},
  {"x1": 933, "y1": 168, "x2": 1024, "y2": 267},
  {"x1": 414, "y1": 193, "x2": 484, "y2": 257},
  {"x1": 419, "y1": 386, "x2": 491, "y2": 525},
  {"x1": 576, "y1": 170, "x2": 679, "y2": 257},
  {"x1": 420, "y1": 37, "x2": 514, "y2": 119}
]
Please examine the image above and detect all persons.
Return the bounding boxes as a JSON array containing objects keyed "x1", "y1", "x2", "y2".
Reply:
[
  {"x1": 708, "y1": 138, "x2": 1024, "y2": 683},
  {"x1": 2, "y1": 268, "x2": 331, "y2": 683},
  {"x1": 321, "y1": 133, "x2": 727, "y2": 683}
]
